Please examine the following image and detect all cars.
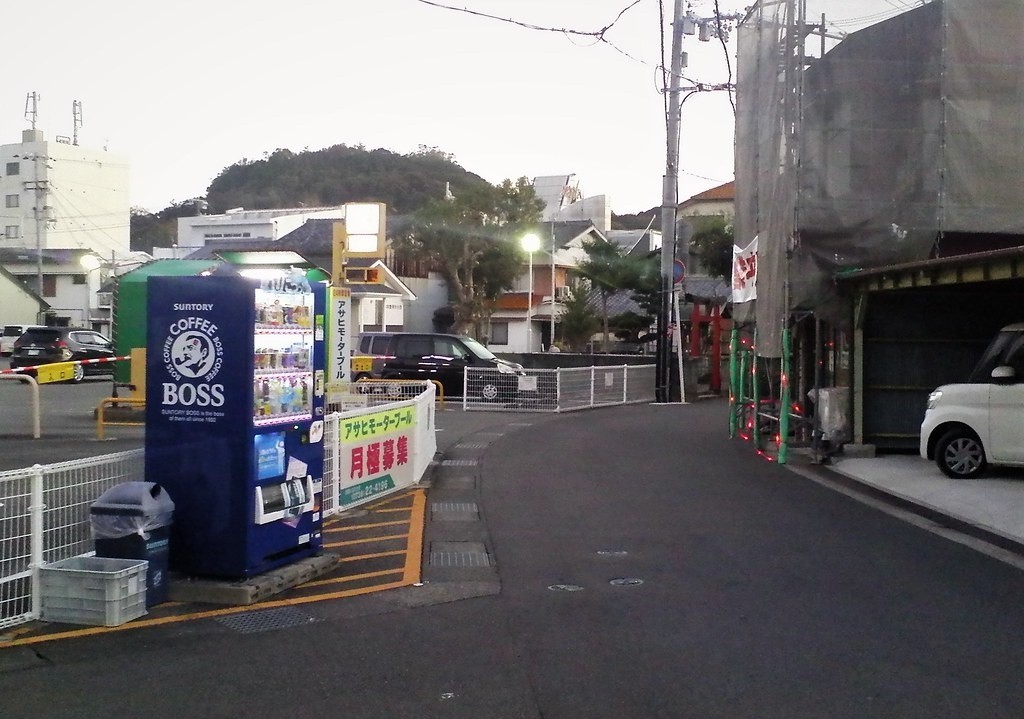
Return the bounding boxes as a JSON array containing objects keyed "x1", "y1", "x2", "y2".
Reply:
[
  {"x1": 920, "y1": 325, "x2": 1024, "y2": 479},
  {"x1": 9, "y1": 326, "x2": 116, "y2": 384},
  {"x1": 1, "y1": 325, "x2": 48, "y2": 357}
]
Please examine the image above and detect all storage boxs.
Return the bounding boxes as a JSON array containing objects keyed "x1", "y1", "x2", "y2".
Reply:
[{"x1": 38, "y1": 555, "x2": 149, "y2": 627}]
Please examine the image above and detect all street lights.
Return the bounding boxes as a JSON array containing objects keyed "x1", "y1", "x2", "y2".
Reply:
[{"x1": 521, "y1": 233, "x2": 540, "y2": 353}]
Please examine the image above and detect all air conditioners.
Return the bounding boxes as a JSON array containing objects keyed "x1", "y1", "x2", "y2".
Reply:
[{"x1": 557, "y1": 286, "x2": 570, "y2": 298}]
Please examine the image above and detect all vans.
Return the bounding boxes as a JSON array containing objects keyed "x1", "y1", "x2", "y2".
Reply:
[
  {"x1": 351, "y1": 332, "x2": 462, "y2": 394},
  {"x1": 381, "y1": 333, "x2": 526, "y2": 402}
]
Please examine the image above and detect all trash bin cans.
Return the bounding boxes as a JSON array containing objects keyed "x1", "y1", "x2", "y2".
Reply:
[{"x1": 90, "y1": 503, "x2": 175, "y2": 606}]
